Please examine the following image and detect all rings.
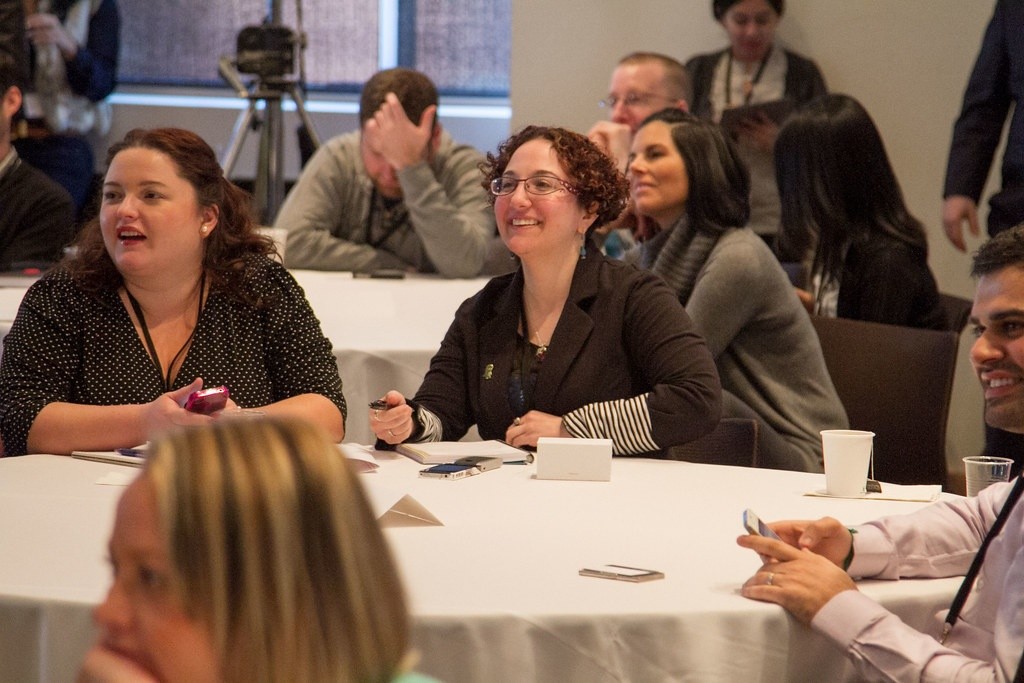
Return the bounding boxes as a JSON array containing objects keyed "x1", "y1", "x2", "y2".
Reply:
[
  {"x1": 389, "y1": 429, "x2": 396, "y2": 437},
  {"x1": 513, "y1": 417, "x2": 520, "y2": 426},
  {"x1": 375, "y1": 410, "x2": 382, "y2": 421},
  {"x1": 766, "y1": 572, "x2": 774, "y2": 585}
]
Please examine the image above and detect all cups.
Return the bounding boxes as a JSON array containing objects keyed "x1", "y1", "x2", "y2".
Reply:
[
  {"x1": 962, "y1": 455, "x2": 1014, "y2": 499},
  {"x1": 820, "y1": 429, "x2": 875, "y2": 497},
  {"x1": 258, "y1": 226, "x2": 290, "y2": 267}
]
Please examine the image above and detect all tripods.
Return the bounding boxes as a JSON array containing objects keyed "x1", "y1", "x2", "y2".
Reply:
[{"x1": 220, "y1": 75, "x2": 324, "y2": 230}]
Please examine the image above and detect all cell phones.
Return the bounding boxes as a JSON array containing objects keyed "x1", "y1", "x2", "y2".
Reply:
[
  {"x1": 419, "y1": 456, "x2": 503, "y2": 480},
  {"x1": 744, "y1": 509, "x2": 782, "y2": 541},
  {"x1": 352, "y1": 268, "x2": 407, "y2": 280},
  {"x1": 184, "y1": 387, "x2": 229, "y2": 415}
]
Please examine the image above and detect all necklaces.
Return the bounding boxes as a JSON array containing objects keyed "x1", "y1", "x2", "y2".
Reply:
[
  {"x1": 725, "y1": 48, "x2": 771, "y2": 106},
  {"x1": 524, "y1": 294, "x2": 551, "y2": 359}
]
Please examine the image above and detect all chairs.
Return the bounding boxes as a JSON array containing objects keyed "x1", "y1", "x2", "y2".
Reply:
[
  {"x1": 811, "y1": 314, "x2": 961, "y2": 493},
  {"x1": 664, "y1": 418, "x2": 759, "y2": 466}
]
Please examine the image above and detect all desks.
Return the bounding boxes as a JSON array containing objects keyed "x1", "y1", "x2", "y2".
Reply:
[
  {"x1": 0, "y1": 440, "x2": 981, "y2": 683},
  {"x1": 0, "y1": 266, "x2": 497, "y2": 446}
]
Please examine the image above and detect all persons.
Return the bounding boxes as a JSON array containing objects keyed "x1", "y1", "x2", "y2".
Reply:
[
  {"x1": 272, "y1": 68, "x2": 496, "y2": 279},
  {"x1": 0, "y1": 48, "x2": 78, "y2": 274},
  {"x1": 0, "y1": 127, "x2": 348, "y2": 457},
  {"x1": 0, "y1": 0, "x2": 122, "y2": 204},
  {"x1": 941, "y1": 0, "x2": 1024, "y2": 253},
  {"x1": 683, "y1": 0, "x2": 831, "y2": 297},
  {"x1": 738, "y1": 220, "x2": 1024, "y2": 683},
  {"x1": 76, "y1": 416, "x2": 438, "y2": 683},
  {"x1": 616, "y1": 108, "x2": 850, "y2": 474},
  {"x1": 586, "y1": 52, "x2": 695, "y2": 253},
  {"x1": 370, "y1": 124, "x2": 722, "y2": 459},
  {"x1": 772, "y1": 94, "x2": 946, "y2": 329}
]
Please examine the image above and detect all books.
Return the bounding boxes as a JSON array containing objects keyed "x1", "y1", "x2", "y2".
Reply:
[{"x1": 396, "y1": 438, "x2": 534, "y2": 465}]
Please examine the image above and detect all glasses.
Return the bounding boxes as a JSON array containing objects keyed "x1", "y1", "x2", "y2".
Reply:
[
  {"x1": 600, "y1": 90, "x2": 680, "y2": 110},
  {"x1": 489, "y1": 177, "x2": 585, "y2": 196}
]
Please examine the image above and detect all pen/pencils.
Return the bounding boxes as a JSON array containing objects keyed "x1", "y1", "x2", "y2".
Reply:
[
  {"x1": 369, "y1": 399, "x2": 397, "y2": 411},
  {"x1": 114, "y1": 448, "x2": 149, "y2": 458}
]
